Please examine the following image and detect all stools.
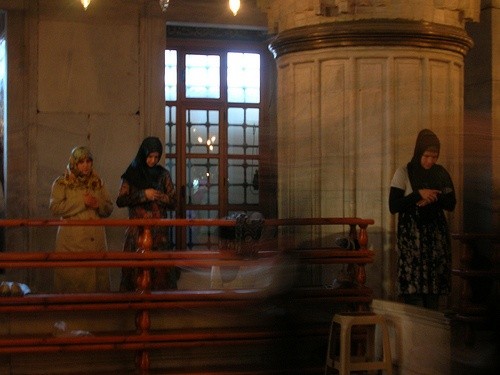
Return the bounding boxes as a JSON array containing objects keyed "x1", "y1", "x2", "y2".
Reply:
[{"x1": 325, "y1": 315, "x2": 392, "y2": 375}]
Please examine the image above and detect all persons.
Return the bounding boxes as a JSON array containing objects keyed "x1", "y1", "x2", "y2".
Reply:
[
  {"x1": 116, "y1": 136, "x2": 176, "y2": 291},
  {"x1": 49, "y1": 145, "x2": 114, "y2": 294},
  {"x1": 389, "y1": 129, "x2": 457, "y2": 310}
]
